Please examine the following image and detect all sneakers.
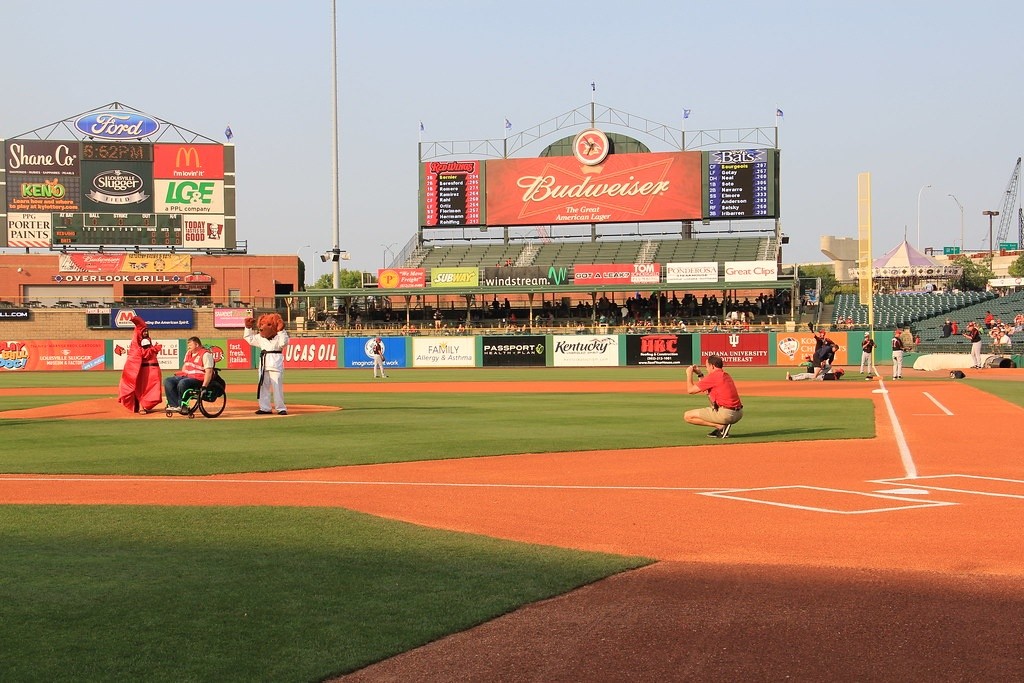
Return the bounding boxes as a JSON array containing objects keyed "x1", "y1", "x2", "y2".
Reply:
[
  {"x1": 720, "y1": 424, "x2": 730, "y2": 438},
  {"x1": 707, "y1": 430, "x2": 729, "y2": 438}
]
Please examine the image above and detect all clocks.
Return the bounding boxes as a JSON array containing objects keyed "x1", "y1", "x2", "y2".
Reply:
[{"x1": 571, "y1": 128, "x2": 609, "y2": 166}]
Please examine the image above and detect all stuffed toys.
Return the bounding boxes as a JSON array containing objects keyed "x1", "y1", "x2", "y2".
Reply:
[{"x1": 243, "y1": 313, "x2": 289, "y2": 415}]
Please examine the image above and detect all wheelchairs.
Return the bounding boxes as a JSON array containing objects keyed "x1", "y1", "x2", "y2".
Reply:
[{"x1": 162, "y1": 367, "x2": 227, "y2": 419}]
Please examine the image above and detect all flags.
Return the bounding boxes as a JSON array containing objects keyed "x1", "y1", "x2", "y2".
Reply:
[
  {"x1": 505, "y1": 119, "x2": 512, "y2": 129},
  {"x1": 777, "y1": 108, "x2": 783, "y2": 116},
  {"x1": 684, "y1": 109, "x2": 690, "y2": 119},
  {"x1": 420, "y1": 122, "x2": 424, "y2": 130}
]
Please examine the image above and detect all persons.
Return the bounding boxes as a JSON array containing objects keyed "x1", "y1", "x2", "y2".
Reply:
[
  {"x1": 324, "y1": 298, "x2": 530, "y2": 335},
  {"x1": 808, "y1": 288, "x2": 816, "y2": 305},
  {"x1": 785, "y1": 322, "x2": 844, "y2": 381},
  {"x1": 495, "y1": 257, "x2": 512, "y2": 267},
  {"x1": 916, "y1": 335, "x2": 920, "y2": 344},
  {"x1": 891, "y1": 330, "x2": 907, "y2": 380},
  {"x1": 963, "y1": 322, "x2": 982, "y2": 369},
  {"x1": 118, "y1": 315, "x2": 167, "y2": 415},
  {"x1": 165, "y1": 337, "x2": 214, "y2": 413},
  {"x1": 178, "y1": 293, "x2": 184, "y2": 304},
  {"x1": 542, "y1": 292, "x2": 791, "y2": 333},
  {"x1": 860, "y1": 332, "x2": 877, "y2": 374},
  {"x1": 683, "y1": 355, "x2": 744, "y2": 438},
  {"x1": 837, "y1": 315, "x2": 854, "y2": 324},
  {"x1": 974, "y1": 311, "x2": 1024, "y2": 346},
  {"x1": 943, "y1": 318, "x2": 958, "y2": 338},
  {"x1": 373, "y1": 338, "x2": 389, "y2": 378}
]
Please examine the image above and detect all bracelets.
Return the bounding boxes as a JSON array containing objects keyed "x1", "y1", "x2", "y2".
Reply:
[{"x1": 698, "y1": 374, "x2": 703, "y2": 377}]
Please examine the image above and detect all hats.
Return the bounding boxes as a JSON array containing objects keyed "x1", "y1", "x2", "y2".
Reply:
[
  {"x1": 839, "y1": 369, "x2": 844, "y2": 374},
  {"x1": 806, "y1": 355, "x2": 810, "y2": 359},
  {"x1": 969, "y1": 323, "x2": 973, "y2": 327},
  {"x1": 895, "y1": 330, "x2": 902, "y2": 334},
  {"x1": 376, "y1": 338, "x2": 381, "y2": 341},
  {"x1": 864, "y1": 334, "x2": 869, "y2": 338},
  {"x1": 820, "y1": 330, "x2": 825, "y2": 335}
]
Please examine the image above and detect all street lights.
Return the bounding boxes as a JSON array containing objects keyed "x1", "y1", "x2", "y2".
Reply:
[
  {"x1": 982, "y1": 210, "x2": 999, "y2": 273},
  {"x1": 948, "y1": 193, "x2": 964, "y2": 254},
  {"x1": 917, "y1": 184, "x2": 932, "y2": 252},
  {"x1": 380, "y1": 244, "x2": 395, "y2": 268},
  {"x1": 383, "y1": 243, "x2": 398, "y2": 269},
  {"x1": 312, "y1": 251, "x2": 319, "y2": 286}
]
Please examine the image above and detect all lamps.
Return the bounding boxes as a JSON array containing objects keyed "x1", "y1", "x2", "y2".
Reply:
[{"x1": 17, "y1": 267, "x2": 22, "y2": 272}]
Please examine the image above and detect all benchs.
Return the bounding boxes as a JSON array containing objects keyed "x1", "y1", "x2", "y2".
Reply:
[
  {"x1": 830, "y1": 293, "x2": 1023, "y2": 353},
  {"x1": 394, "y1": 236, "x2": 777, "y2": 282}
]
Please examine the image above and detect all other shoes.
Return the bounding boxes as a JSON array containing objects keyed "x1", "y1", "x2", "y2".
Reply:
[
  {"x1": 138, "y1": 410, "x2": 146, "y2": 415},
  {"x1": 787, "y1": 372, "x2": 793, "y2": 381},
  {"x1": 166, "y1": 406, "x2": 182, "y2": 412},
  {"x1": 894, "y1": 376, "x2": 897, "y2": 380},
  {"x1": 899, "y1": 376, "x2": 903, "y2": 379}
]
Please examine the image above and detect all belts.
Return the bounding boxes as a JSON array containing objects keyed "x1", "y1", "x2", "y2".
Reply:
[{"x1": 720, "y1": 405, "x2": 743, "y2": 411}]
